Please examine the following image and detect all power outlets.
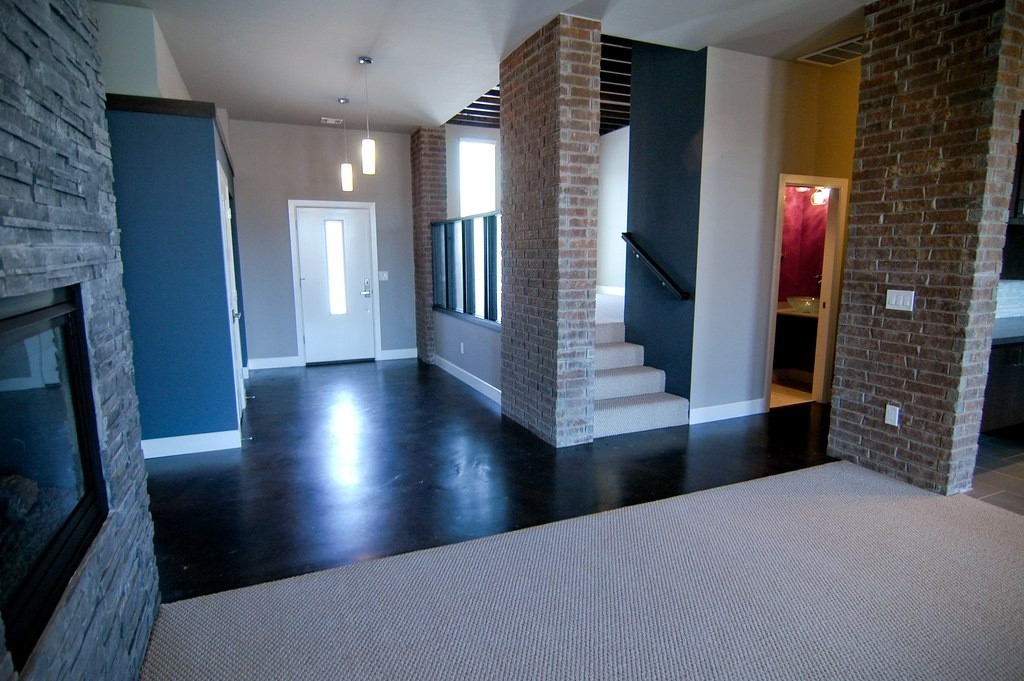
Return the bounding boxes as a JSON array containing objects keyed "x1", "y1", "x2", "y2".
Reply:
[{"x1": 884, "y1": 405, "x2": 899, "y2": 426}]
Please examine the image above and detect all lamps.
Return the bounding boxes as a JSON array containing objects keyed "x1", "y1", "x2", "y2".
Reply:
[
  {"x1": 358, "y1": 56, "x2": 375, "y2": 174},
  {"x1": 338, "y1": 98, "x2": 354, "y2": 191}
]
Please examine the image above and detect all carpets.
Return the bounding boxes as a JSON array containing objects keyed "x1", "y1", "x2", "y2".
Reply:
[{"x1": 138, "y1": 460, "x2": 1024, "y2": 681}]
